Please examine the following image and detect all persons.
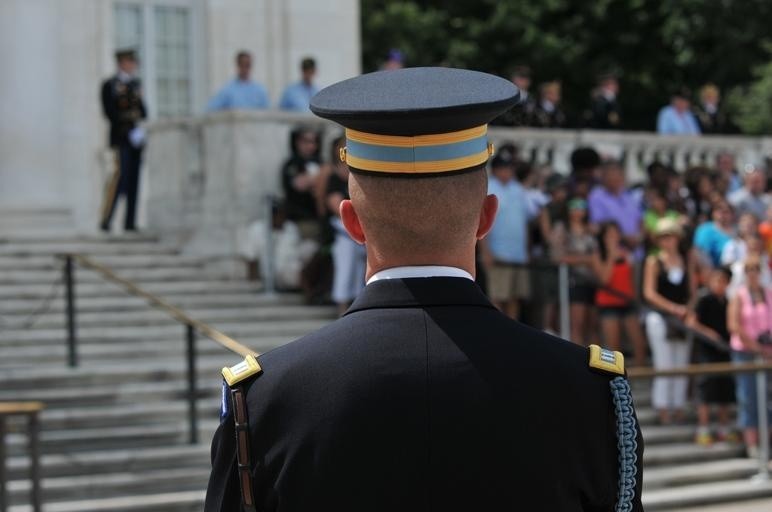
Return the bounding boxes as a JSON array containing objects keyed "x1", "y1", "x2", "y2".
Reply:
[
  {"x1": 99, "y1": 46, "x2": 147, "y2": 230},
  {"x1": 280, "y1": 57, "x2": 318, "y2": 110},
  {"x1": 492, "y1": 65, "x2": 542, "y2": 126},
  {"x1": 199, "y1": 53, "x2": 271, "y2": 110},
  {"x1": 204, "y1": 64, "x2": 643, "y2": 511},
  {"x1": 528, "y1": 76, "x2": 568, "y2": 128},
  {"x1": 281, "y1": 126, "x2": 367, "y2": 318},
  {"x1": 372, "y1": 44, "x2": 403, "y2": 72},
  {"x1": 582, "y1": 74, "x2": 633, "y2": 131},
  {"x1": 475, "y1": 143, "x2": 772, "y2": 458},
  {"x1": 693, "y1": 83, "x2": 743, "y2": 134},
  {"x1": 656, "y1": 86, "x2": 701, "y2": 134}
]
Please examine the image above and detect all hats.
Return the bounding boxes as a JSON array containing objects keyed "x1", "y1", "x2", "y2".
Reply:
[
  {"x1": 113, "y1": 46, "x2": 139, "y2": 63},
  {"x1": 309, "y1": 67, "x2": 520, "y2": 177}
]
